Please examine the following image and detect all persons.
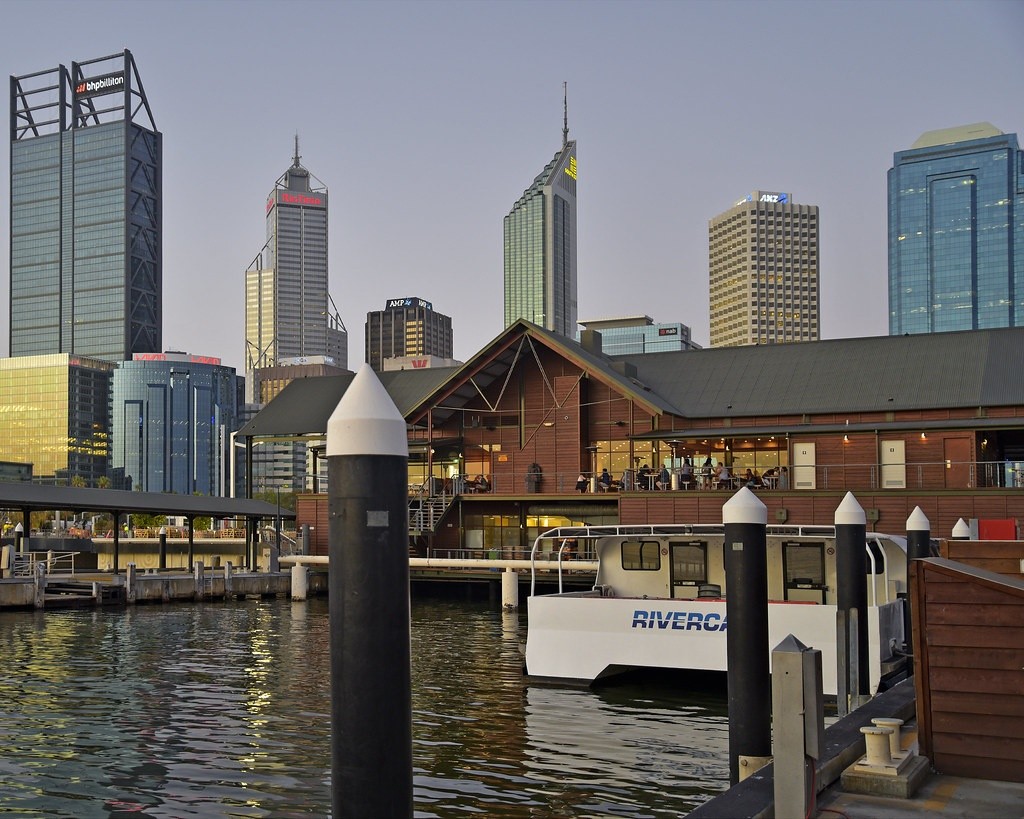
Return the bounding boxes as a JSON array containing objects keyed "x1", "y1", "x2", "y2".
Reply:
[
  {"x1": 459, "y1": 472, "x2": 487, "y2": 493},
  {"x1": 3, "y1": 521, "x2": 15, "y2": 538},
  {"x1": 576, "y1": 457, "x2": 787, "y2": 493},
  {"x1": 122, "y1": 522, "x2": 128, "y2": 537},
  {"x1": 72, "y1": 518, "x2": 94, "y2": 543}
]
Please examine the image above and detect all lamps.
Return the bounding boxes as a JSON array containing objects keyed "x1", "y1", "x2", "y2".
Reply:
[
  {"x1": 842, "y1": 434, "x2": 849, "y2": 441},
  {"x1": 918, "y1": 433, "x2": 929, "y2": 441}
]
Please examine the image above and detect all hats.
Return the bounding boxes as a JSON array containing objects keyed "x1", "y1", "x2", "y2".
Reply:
[{"x1": 123, "y1": 523, "x2": 127, "y2": 525}]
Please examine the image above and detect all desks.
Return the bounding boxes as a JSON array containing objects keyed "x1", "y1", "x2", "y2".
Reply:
[
  {"x1": 693, "y1": 473, "x2": 708, "y2": 490},
  {"x1": 765, "y1": 477, "x2": 779, "y2": 490},
  {"x1": 644, "y1": 474, "x2": 659, "y2": 490},
  {"x1": 652, "y1": 473, "x2": 661, "y2": 490}
]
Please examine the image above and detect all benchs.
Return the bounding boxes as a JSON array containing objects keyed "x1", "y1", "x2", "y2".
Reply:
[
  {"x1": 469, "y1": 488, "x2": 475, "y2": 494},
  {"x1": 616, "y1": 486, "x2": 623, "y2": 492},
  {"x1": 662, "y1": 482, "x2": 670, "y2": 490},
  {"x1": 755, "y1": 484, "x2": 761, "y2": 489},
  {"x1": 634, "y1": 482, "x2": 644, "y2": 490},
  {"x1": 713, "y1": 482, "x2": 720, "y2": 489},
  {"x1": 728, "y1": 477, "x2": 737, "y2": 490},
  {"x1": 578, "y1": 488, "x2": 583, "y2": 494},
  {"x1": 603, "y1": 487, "x2": 610, "y2": 493},
  {"x1": 683, "y1": 481, "x2": 690, "y2": 490}
]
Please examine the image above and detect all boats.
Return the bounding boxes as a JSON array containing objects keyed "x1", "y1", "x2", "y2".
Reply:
[{"x1": 522, "y1": 522, "x2": 907, "y2": 697}]
